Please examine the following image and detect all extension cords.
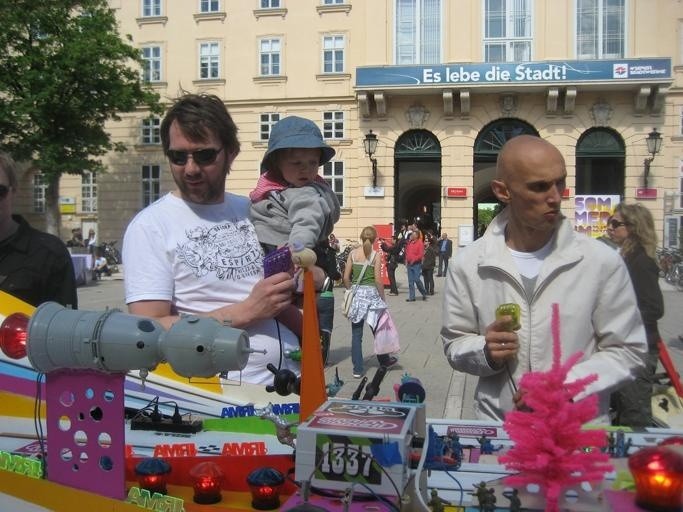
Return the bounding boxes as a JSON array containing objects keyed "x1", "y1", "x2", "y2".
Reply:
[{"x1": 130, "y1": 416, "x2": 202, "y2": 433}]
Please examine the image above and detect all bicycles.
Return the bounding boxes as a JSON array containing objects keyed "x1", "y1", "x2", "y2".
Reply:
[
  {"x1": 329, "y1": 239, "x2": 357, "y2": 288},
  {"x1": 100, "y1": 242, "x2": 120, "y2": 263},
  {"x1": 654, "y1": 247, "x2": 683, "y2": 293}
]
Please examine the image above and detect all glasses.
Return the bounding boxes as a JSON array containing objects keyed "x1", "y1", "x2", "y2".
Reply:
[
  {"x1": 166, "y1": 146, "x2": 225, "y2": 166},
  {"x1": 607, "y1": 219, "x2": 628, "y2": 228},
  {"x1": 0, "y1": 183, "x2": 13, "y2": 200}
]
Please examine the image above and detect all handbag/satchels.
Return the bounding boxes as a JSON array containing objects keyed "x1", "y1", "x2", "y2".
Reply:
[{"x1": 341, "y1": 289, "x2": 356, "y2": 318}]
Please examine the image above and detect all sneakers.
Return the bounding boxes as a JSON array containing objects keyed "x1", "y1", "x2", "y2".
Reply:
[
  {"x1": 385, "y1": 274, "x2": 447, "y2": 302},
  {"x1": 380, "y1": 356, "x2": 397, "y2": 369},
  {"x1": 352, "y1": 370, "x2": 362, "y2": 377}
]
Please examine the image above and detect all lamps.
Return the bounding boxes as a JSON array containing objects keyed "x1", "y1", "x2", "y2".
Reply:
[
  {"x1": 643, "y1": 127, "x2": 663, "y2": 188},
  {"x1": 362, "y1": 128, "x2": 379, "y2": 189}
]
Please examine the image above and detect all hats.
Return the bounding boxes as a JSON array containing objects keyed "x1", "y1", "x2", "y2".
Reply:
[{"x1": 260, "y1": 115, "x2": 336, "y2": 173}]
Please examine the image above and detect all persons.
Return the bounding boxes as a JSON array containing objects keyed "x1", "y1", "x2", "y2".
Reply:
[
  {"x1": 71, "y1": 226, "x2": 110, "y2": 279},
  {"x1": 607, "y1": 201, "x2": 664, "y2": 427},
  {"x1": 0, "y1": 151, "x2": 77, "y2": 311},
  {"x1": 122, "y1": 81, "x2": 302, "y2": 388},
  {"x1": 248, "y1": 115, "x2": 340, "y2": 369},
  {"x1": 440, "y1": 134, "x2": 648, "y2": 426},
  {"x1": 595, "y1": 215, "x2": 619, "y2": 250},
  {"x1": 330, "y1": 212, "x2": 452, "y2": 379}
]
